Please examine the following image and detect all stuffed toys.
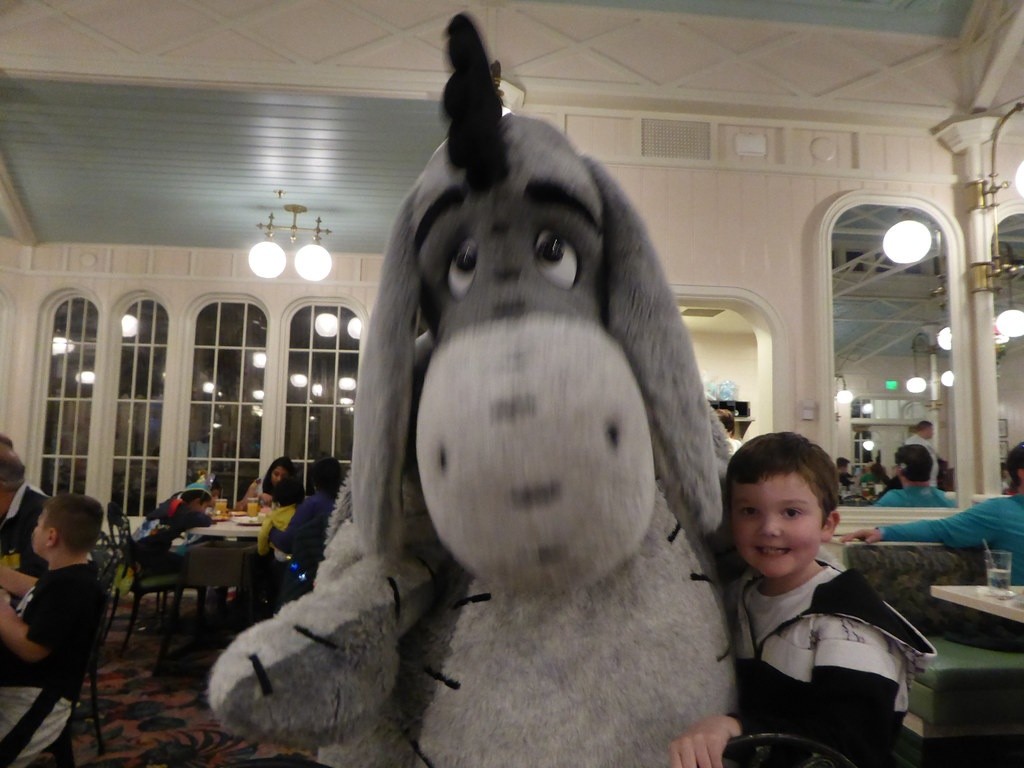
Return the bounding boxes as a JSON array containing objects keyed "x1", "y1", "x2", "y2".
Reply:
[{"x1": 207, "y1": 14, "x2": 737, "y2": 767}]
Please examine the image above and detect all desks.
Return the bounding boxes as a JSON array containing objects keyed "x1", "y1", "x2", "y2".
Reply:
[
  {"x1": 183, "y1": 509, "x2": 265, "y2": 633},
  {"x1": 930, "y1": 583, "x2": 1024, "y2": 655}
]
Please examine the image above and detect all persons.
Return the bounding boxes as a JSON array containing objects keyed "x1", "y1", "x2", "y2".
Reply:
[
  {"x1": 0, "y1": 433, "x2": 349, "y2": 768},
  {"x1": 662, "y1": 431, "x2": 939, "y2": 768},
  {"x1": 832, "y1": 419, "x2": 1024, "y2": 656},
  {"x1": 714, "y1": 409, "x2": 743, "y2": 459}
]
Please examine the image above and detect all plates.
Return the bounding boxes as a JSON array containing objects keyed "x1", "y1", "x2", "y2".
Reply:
[{"x1": 233, "y1": 516, "x2": 262, "y2": 525}]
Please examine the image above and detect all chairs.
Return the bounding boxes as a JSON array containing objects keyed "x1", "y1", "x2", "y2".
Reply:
[
  {"x1": 0, "y1": 500, "x2": 331, "y2": 768},
  {"x1": 696, "y1": 731, "x2": 858, "y2": 768}
]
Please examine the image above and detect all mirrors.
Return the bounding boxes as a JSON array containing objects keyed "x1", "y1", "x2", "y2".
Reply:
[{"x1": 815, "y1": 186, "x2": 1022, "y2": 512}]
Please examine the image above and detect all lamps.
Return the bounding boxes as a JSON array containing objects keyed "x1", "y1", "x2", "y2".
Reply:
[
  {"x1": 246, "y1": 203, "x2": 332, "y2": 283},
  {"x1": 834, "y1": 374, "x2": 854, "y2": 403}
]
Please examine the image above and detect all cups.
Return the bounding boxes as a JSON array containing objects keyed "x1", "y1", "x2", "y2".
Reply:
[
  {"x1": 246, "y1": 496, "x2": 259, "y2": 516},
  {"x1": 984, "y1": 549, "x2": 1012, "y2": 596},
  {"x1": 214, "y1": 498, "x2": 228, "y2": 515}
]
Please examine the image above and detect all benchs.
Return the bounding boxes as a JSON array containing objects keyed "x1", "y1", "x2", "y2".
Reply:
[{"x1": 810, "y1": 505, "x2": 1024, "y2": 768}]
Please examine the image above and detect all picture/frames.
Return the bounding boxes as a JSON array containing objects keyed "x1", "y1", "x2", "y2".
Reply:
[
  {"x1": 997, "y1": 419, "x2": 1009, "y2": 438},
  {"x1": 999, "y1": 441, "x2": 1009, "y2": 463}
]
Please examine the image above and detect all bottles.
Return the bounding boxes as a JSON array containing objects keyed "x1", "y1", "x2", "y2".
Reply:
[{"x1": 860, "y1": 482, "x2": 869, "y2": 497}]
